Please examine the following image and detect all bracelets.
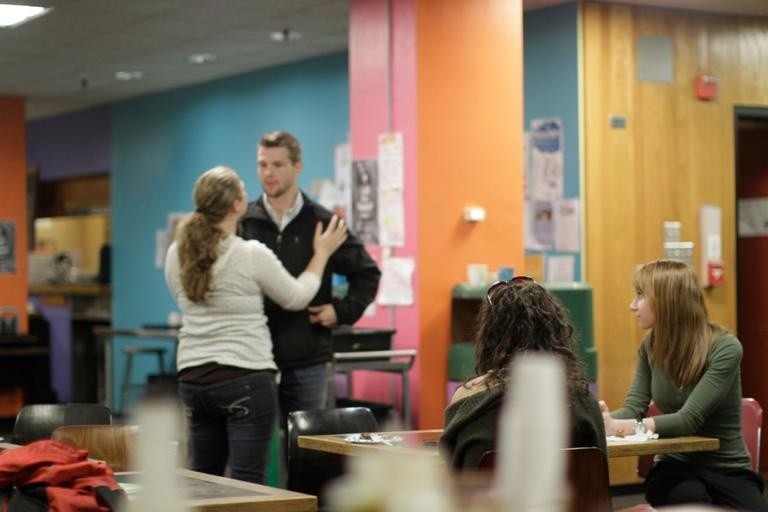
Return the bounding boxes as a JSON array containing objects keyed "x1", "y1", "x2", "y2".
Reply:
[{"x1": 634, "y1": 417, "x2": 646, "y2": 434}]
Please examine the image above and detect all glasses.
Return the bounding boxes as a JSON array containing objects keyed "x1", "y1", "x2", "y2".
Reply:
[{"x1": 485, "y1": 274, "x2": 533, "y2": 305}]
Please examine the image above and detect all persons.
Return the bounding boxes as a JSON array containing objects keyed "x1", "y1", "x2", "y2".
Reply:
[
  {"x1": 163, "y1": 162, "x2": 350, "y2": 489},
  {"x1": 436, "y1": 274, "x2": 614, "y2": 511},
  {"x1": 219, "y1": 130, "x2": 381, "y2": 488},
  {"x1": 590, "y1": 259, "x2": 768, "y2": 511}
]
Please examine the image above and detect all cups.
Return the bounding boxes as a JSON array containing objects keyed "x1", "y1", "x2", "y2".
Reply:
[
  {"x1": 468, "y1": 263, "x2": 488, "y2": 287},
  {"x1": 497, "y1": 266, "x2": 514, "y2": 282}
]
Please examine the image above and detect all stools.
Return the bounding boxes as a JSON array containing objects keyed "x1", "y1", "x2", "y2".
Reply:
[
  {"x1": 93, "y1": 328, "x2": 179, "y2": 413},
  {"x1": 119, "y1": 346, "x2": 167, "y2": 416}
]
